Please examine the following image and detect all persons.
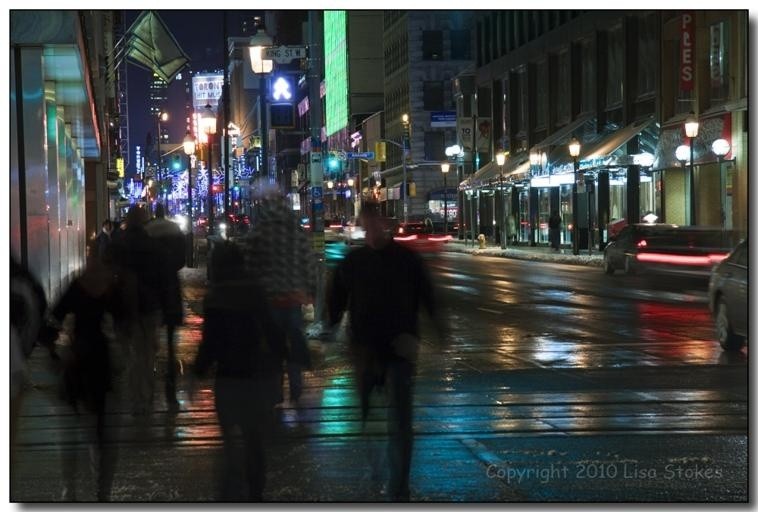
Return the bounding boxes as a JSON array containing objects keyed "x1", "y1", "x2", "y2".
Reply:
[
  {"x1": 547, "y1": 207, "x2": 562, "y2": 252},
  {"x1": 45, "y1": 233, "x2": 151, "y2": 501},
  {"x1": 315, "y1": 191, "x2": 449, "y2": 501},
  {"x1": 89, "y1": 200, "x2": 191, "y2": 366},
  {"x1": 185, "y1": 238, "x2": 294, "y2": 499},
  {"x1": 244, "y1": 191, "x2": 318, "y2": 405}
]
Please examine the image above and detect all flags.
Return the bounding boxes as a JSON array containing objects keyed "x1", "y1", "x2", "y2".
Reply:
[
  {"x1": 126, "y1": 11, "x2": 185, "y2": 67},
  {"x1": 123, "y1": 48, "x2": 191, "y2": 86},
  {"x1": 127, "y1": 34, "x2": 191, "y2": 78}
]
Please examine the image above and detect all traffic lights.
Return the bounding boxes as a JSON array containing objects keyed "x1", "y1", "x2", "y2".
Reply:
[
  {"x1": 171, "y1": 153, "x2": 183, "y2": 171},
  {"x1": 403, "y1": 121, "x2": 412, "y2": 138},
  {"x1": 268, "y1": 71, "x2": 297, "y2": 105}
]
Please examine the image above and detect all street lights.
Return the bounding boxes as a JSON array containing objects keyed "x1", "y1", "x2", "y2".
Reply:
[
  {"x1": 439, "y1": 159, "x2": 449, "y2": 235},
  {"x1": 496, "y1": 146, "x2": 509, "y2": 250},
  {"x1": 183, "y1": 128, "x2": 197, "y2": 248},
  {"x1": 201, "y1": 97, "x2": 215, "y2": 242},
  {"x1": 685, "y1": 106, "x2": 701, "y2": 224},
  {"x1": 570, "y1": 135, "x2": 585, "y2": 259},
  {"x1": 157, "y1": 109, "x2": 170, "y2": 218},
  {"x1": 248, "y1": 27, "x2": 277, "y2": 190},
  {"x1": 444, "y1": 144, "x2": 464, "y2": 239}
]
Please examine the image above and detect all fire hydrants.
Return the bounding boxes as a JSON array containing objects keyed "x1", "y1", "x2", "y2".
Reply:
[{"x1": 477, "y1": 232, "x2": 487, "y2": 249}]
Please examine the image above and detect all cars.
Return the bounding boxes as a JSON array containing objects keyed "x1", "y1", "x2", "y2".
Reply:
[
  {"x1": 454, "y1": 209, "x2": 659, "y2": 249},
  {"x1": 173, "y1": 209, "x2": 453, "y2": 270},
  {"x1": 604, "y1": 222, "x2": 748, "y2": 351}
]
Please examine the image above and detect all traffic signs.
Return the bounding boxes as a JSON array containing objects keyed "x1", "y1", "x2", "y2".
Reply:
[{"x1": 347, "y1": 152, "x2": 374, "y2": 160}]
